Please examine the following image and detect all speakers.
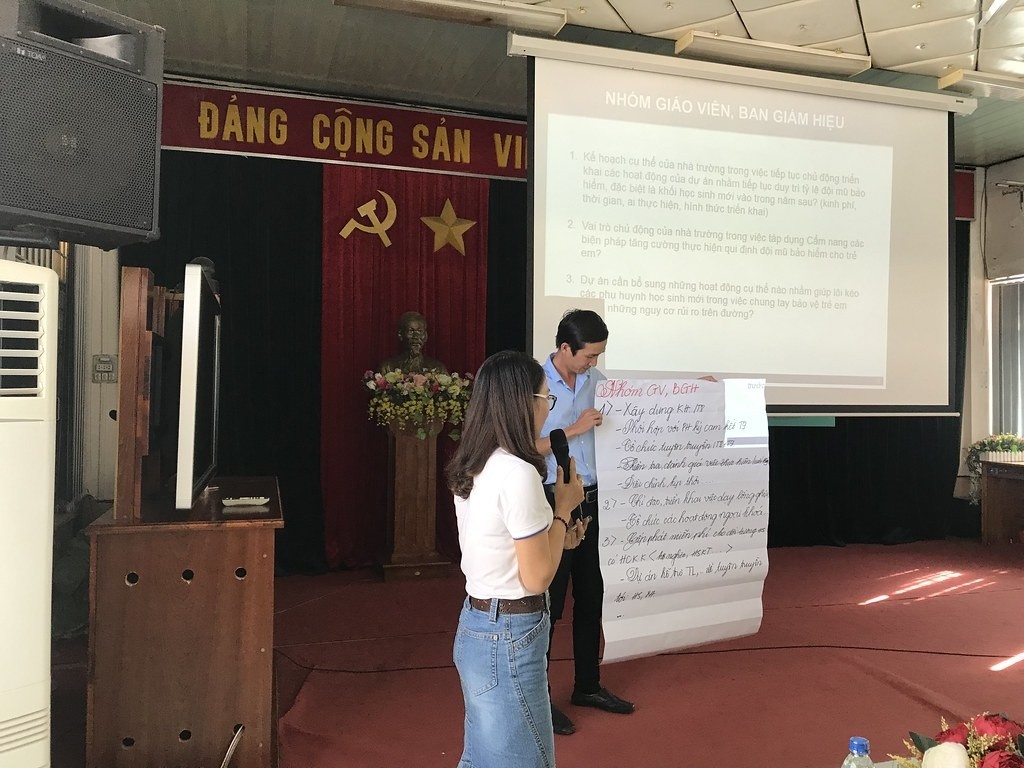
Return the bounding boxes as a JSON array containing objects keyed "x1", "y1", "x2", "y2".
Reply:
[{"x1": 0, "y1": 30, "x2": 165, "y2": 256}]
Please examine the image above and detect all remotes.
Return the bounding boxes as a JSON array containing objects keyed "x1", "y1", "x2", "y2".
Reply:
[{"x1": 221, "y1": 496, "x2": 270, "y2": 507}]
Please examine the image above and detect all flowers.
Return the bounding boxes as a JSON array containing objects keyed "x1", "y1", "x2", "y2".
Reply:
[
  {"x1": 963, "y1": 432, "x2": 1024, "y2": 506},
  {"x1": 361, "y1": 367, "x2": 475, "y2": 441}
]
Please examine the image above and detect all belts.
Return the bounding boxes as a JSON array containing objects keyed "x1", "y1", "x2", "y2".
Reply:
[
  {"x1": 470, "y1": 593, "x2": 546, "y2": 615},
  {"x1": 583, "y1": 489, "x2": 598, "y2": 503}
]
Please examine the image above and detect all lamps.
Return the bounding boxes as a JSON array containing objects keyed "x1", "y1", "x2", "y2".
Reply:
[
  {"x1": 937, "y1": 69, "x2": 1024, "y2": 102},
  {"x1": 332, "y1": 0, "x2": 567, "y2": 36},
  {"x1": 674, "y1": 29, "x2": 873, "y2": 79}
]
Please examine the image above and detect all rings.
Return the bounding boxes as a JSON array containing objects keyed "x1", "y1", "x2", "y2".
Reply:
[{"x1": 581, "y1": 535, "x2": 585, "y2": 540}]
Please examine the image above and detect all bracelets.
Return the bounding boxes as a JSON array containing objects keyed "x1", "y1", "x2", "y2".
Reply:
[{"x1": 554, "y1": 516, "x2": 568, "y2": 532}]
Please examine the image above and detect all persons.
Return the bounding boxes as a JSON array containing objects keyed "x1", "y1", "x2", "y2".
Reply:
[
  {"x1": 374, "y1": 311, "x2": 451, "y2": 377},
  {"x1": 536, "y1": 309, "x2": 718, "y2": 735},
  {"x1": 441, "y1": 352, "x2": 588, "y2": 768}
]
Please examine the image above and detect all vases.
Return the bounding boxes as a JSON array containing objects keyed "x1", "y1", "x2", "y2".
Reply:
[{"x1": 980, "y1": 451, "x2": 1024, "y2": 462}]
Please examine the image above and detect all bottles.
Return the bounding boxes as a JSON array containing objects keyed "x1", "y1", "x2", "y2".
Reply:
[{"x1": 840, "y1": 736, "x2": 876, "y2": 768}]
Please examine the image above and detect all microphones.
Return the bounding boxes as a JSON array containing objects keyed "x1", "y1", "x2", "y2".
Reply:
[{"x1": 549, "y1": 428, "x2": 583, "y2": 523}]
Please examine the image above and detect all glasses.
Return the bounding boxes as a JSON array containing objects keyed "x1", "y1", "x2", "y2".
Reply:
[{"x1": 531, "y1": 392, "x2": 557, "y2": 411}]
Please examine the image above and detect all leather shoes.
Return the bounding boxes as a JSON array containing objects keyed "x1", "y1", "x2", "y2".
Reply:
[
  {"x1": 551, "y1": 705, "x2": 575, "y2": 735},
  {"x1": 572, "y1": 685, "x2": 634, "y2": 714}
]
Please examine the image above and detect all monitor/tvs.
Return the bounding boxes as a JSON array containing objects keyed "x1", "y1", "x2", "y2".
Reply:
[{"x1": 175, "y1": 263, "x2": 223, "y2": 510}]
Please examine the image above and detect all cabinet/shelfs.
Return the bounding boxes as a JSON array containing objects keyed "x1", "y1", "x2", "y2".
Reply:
[{"x1": 84, "y1": 476, "x2": 284, "y2": 768}]
